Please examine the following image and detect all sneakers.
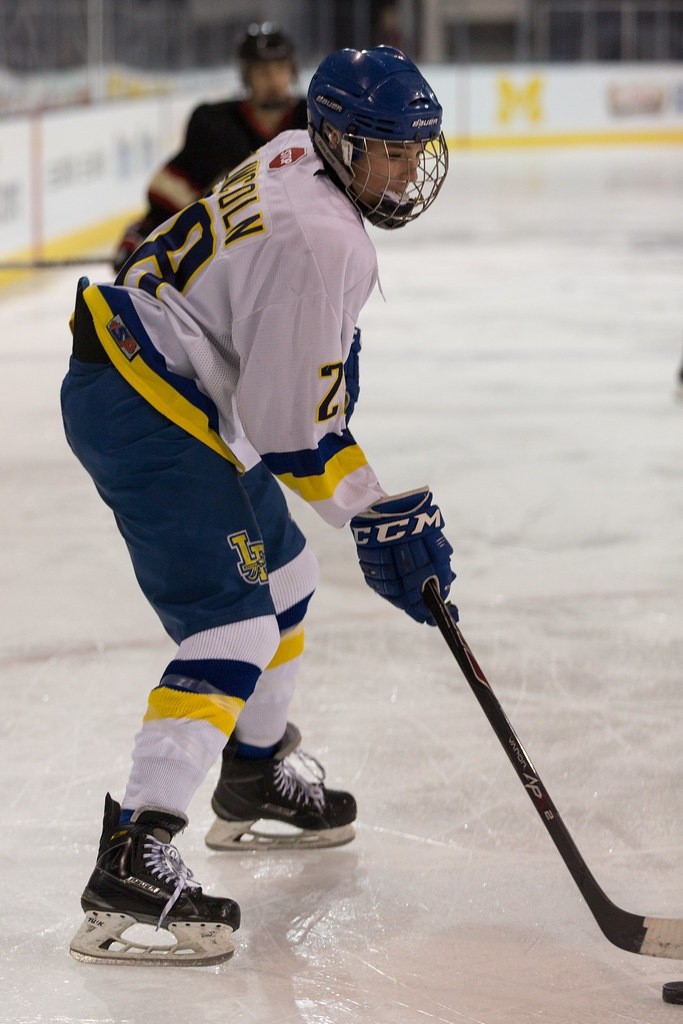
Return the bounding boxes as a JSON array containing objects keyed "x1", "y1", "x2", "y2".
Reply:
[
  {"x1": 206, "y1": 722, "x2": 358, "y2": 852},
  {"x1": 70, "y1": 792, "x2": 240, "y2": 968}
]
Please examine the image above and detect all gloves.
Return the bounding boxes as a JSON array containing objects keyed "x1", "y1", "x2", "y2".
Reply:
[
  {"x1": 345, "y1": 330, "x2": 360, "y2": 424},
  {"x1": 350, "y1": 486, "x2": 459, "y2": 627}
]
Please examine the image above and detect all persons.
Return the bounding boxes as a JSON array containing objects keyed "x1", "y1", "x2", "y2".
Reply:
[
  {"x1": 111, "y1": 23, "x2": 307, "y2": 274},
  {"x1": 61, "y1": 45, "x2": 461, "y2": 968}
]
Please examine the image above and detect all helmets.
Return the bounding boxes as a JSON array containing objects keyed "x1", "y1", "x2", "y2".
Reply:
[
  {"x1": 239, "y1": 21, "x2": 300, "y2": 86},
  {"x1": 306, "y1": 44, "x2": 450, "y2": 230}
]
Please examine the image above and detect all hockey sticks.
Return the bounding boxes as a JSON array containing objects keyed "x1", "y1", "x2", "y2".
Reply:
[{"x1": 439, "y1": 599, "x2": 682, "y2": 961}]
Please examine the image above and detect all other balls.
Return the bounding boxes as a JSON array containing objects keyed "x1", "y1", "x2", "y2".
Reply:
[{"x1": 661, "y1": 980, "x2": 683, "y2": 1005}]
